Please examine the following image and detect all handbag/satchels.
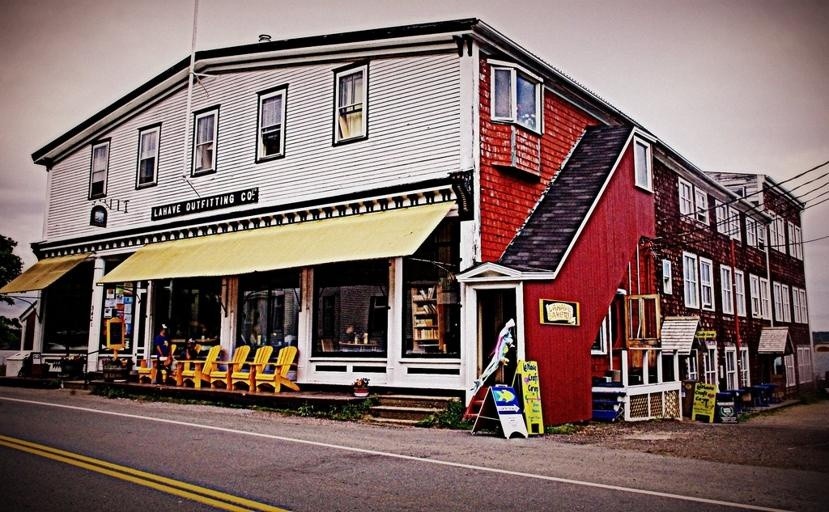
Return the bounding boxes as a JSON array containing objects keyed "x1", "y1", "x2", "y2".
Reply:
[{"x1": 164, "y1": 351, "x2": 173, "y2": 367}]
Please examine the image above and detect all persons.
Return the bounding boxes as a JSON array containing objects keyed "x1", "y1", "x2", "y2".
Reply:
[
  {"x1": 245, "y1": 322, "x2": 263, "y2": 357},
  {"x1": 169, "y1": 336, "x2": 197, "y2": 386},
  {"x1": 154, "y1": 323, "x2": 175, "y2": 384},
  {"x1": 333, "y1": 323, "x2": 357, "y2": 353}
]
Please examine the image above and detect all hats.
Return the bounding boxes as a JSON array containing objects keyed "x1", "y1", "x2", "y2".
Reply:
[
  {"x1": 188, "y1": 337, "x2": 196, "y2": 344},
  {"x1": 161, "y1": 323, "x2": 168, "y2": 329}
]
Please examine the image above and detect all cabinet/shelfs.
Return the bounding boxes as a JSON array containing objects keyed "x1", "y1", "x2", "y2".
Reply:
[{"x1": 409, "y1": 281, "x2": 446, "y2": 354}]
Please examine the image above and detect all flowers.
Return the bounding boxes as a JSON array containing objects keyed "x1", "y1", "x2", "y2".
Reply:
[{"x1": 352, "y1": 378, "x2": 370, "y2": 389}]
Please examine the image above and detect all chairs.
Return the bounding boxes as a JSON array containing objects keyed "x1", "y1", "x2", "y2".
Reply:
[
  {"x1": 138, "y1": 344, "x2": 300, "y2": 395},
  {"x1": 715, "y1": 383, "x2": 780, "y2": 423},
  {"x1": 320, "y1": 338, "x2": 334, "y2": 352}
]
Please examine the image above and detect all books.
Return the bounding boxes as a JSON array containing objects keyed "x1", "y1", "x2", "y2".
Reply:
[{"x1": 413, "y1": 286, "x2": 439, "y2": 340}]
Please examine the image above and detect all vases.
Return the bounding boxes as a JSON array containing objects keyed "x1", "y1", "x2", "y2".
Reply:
[{"x1": 354, "y1": 388, "x2": 369, "y2": 397}]
